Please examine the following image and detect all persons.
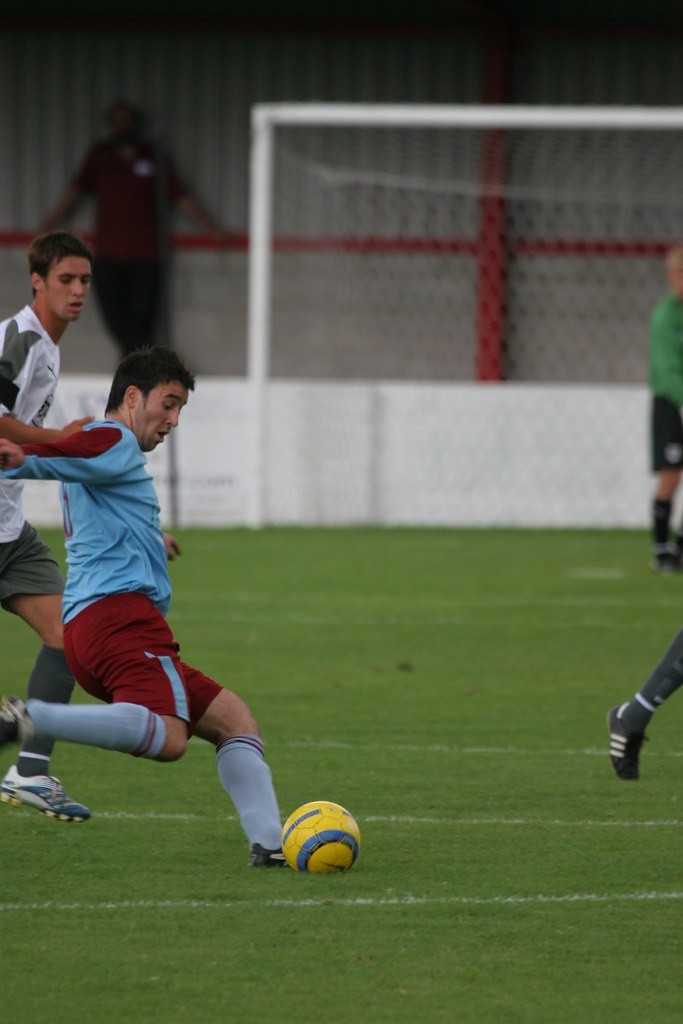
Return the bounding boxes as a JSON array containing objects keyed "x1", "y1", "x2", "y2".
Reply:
[
  {"x1": 0, "y1": 232, "x2": 91, "y2": 818},
  {"x1": 0, "y1": 344, "x2": 292, "y2": 870},
  {"x1": 607, "y1": 631, "x2": 683, "y2": 780},
  {"x1": 645, "y1": 246, "x2": 683, "y2": 572},
  {"x1": 37, "y1": 102, "x2": 235, "y2": 358}
]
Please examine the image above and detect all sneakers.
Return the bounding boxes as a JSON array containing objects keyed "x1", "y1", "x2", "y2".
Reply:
[
  {"x1": 0, "y1": 696, "x2": 31, "y2": 748},
  {"x1": 247, "y1": 843, "x2": 287, "y2": 866},
  {"x1": 607, "y1": 703, "x2": 648, "y2": 780},
  {"x1": 1, "y1": 765, "x2": 92, "y2": 822}
]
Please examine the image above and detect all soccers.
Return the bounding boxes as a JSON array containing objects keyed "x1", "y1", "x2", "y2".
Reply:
[{"x1": 280, "y1": 800, "x2": 361, "y2": 874}]
